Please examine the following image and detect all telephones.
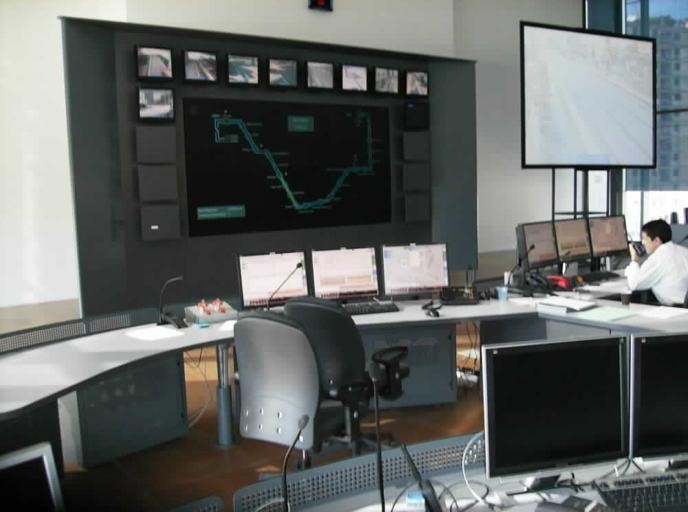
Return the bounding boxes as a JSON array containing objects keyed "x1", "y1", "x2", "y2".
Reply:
[
  {"x1": 524, "y1": 271, "x2": 553, "y2": 293},
  {"x1": 439, "y1": 286, "x2": 479, "y2": 306}
]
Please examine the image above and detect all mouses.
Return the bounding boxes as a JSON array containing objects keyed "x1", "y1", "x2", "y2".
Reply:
[{"x1": 426, "y1": 305, "x2": 440, "y2": 318}]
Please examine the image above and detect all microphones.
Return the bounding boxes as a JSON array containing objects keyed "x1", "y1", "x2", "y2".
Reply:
[
  {"x1": 157, "y1": 272, "x2": 181, "y2": 331},
  {"x1": 504, "y1": 242, "x2": 535, "y2": 296},
  {"x1": 266, "y1": 262, "x2": 304, "y2": 315}
]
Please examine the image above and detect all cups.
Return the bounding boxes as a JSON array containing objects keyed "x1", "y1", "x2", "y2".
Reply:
[
  {"x1": 497, "y1": 286, "x2": 508, "y2": 304},
  {"x1": 503, "y1": 271, "x2": 514, "y2": 285},
  {"x1": 621, "y1": 291, "x2": 632, "y2": 305}
]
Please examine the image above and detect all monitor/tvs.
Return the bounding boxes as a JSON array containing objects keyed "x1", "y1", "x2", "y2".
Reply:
[
  {"x1": 235, "y1": 249, "x2": 307, "y2": 310},
  {"x1": 226, "y1": 52, "x2": 259, "y2": 85},
  {"x1": 139, "y1": 88, "x2": 175, "y2": 120},
  {"x1": 480, "y1": 334, "x2": 631, "y2": 497},
  {"x1": 0, "y1": 440, "x2": 66, "y2": 512},
  {"x1": 184, "y1": 48, "x2": 218, "y2": 81},
  {"x1": 377, "y1": 67, "x2": 398, "y2": 95},
  {"x1": 516, "y1": 222, "x2": 560, "y2": 278},
  {"x1": 585, "y1": 216, "x2": 631, "y2": 271},
  {"x1": 267, "y1": 58, "x2": 299, "y2": 90},
  {"x1": 373, "y1": 243, "x2": 450, "y2": 309},
  {"x1": 310, "y1": 245, "x2": 382, "y2": 307},
  {"x1": 630, "y1": 332, "x2": 688, "y2": 471},
  {"x1": 552, "y1": 219, "x2": 592, "y2": 272},
  {"x1": 408, "y1": 71, "x2": 428, "y2": 97},
  {"x1": 344, "y1": 66, "x2": 369, "y2": 94},
  {"x1": 307, "y1": 60, "x2": 333, "y2": 90},
  {"x1": 135, "y1": 44, "x2": 174, "y2": 80}
]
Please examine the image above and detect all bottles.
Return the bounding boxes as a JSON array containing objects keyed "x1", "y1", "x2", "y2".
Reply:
[{"x1": 464, "y1": 264, "x2": 475, "y2": 298}]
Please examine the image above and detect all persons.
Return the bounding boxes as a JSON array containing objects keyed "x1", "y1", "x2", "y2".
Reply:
[{"x1": 624, "y1": 220, "x2": 688, "y2": 308}]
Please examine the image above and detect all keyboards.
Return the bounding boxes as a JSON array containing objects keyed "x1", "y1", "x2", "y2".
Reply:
[
  {"x1": 591, "y1": 471, "x2": 688, "y2": 512},
  {"x1": 339, "y1": 301, "x2": 400, "y2": 316}
]
